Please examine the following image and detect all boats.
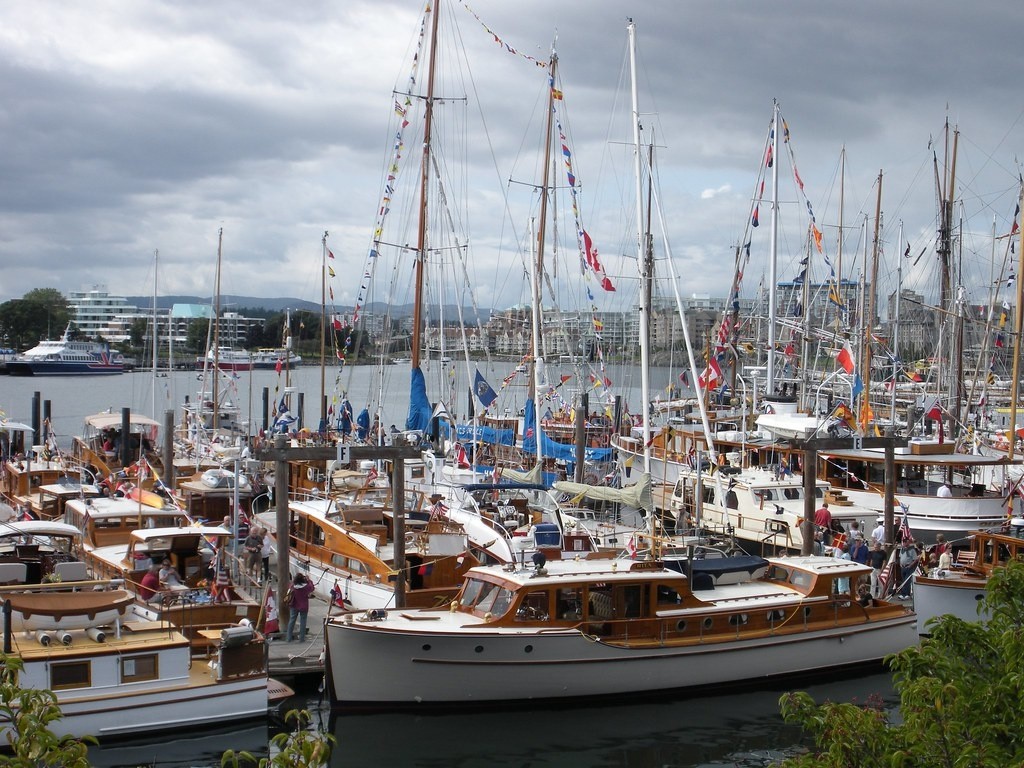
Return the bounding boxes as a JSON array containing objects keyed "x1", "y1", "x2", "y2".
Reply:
[
  {"x1": 6, "y1": 322, "x2": 125, "y2": 376},
  {"x1": 197, "y1": 345, "x2": 301, "y2": 370},
  {"x1": 0, "y1": 419, "x2": 272, "y2": 741}
]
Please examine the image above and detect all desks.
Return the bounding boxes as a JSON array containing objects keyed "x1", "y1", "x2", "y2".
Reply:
[
  {"x1": 196, "y1": 630, "x2": 222, "y2": 649},
  {"x1": 405, "y1": 519, "x2": 428, "y2": 549}
]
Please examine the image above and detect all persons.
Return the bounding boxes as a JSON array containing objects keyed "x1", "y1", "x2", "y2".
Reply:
[
  {"x1": 286, "y1": 573, "x2": 315, "y2": 642},
  {"x1": 141, "y1": 514, "x2": 271, "y2": 605},
  {"x1": 371, "y1": 416, "x2": 400, "y2": 446},
  {"x1": 675, "y1": 505, "x2": 691, "y2": 536},
  {"x1": 814, "y1": 480, "x2": 954, "y2": 608},
  {"x1": 84, "y1": 437, "x2": 165, "y2": 498},
  {"x1": 542, "y1": 407, "x2": 611, "y2": 448},
  {"x1": 23, "y1": 501, "x2": 40, "y2": 520}
]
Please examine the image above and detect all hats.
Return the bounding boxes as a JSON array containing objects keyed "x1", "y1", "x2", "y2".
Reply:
[
  {"x1": 876, "y1": 518, "x2": 885, "y2": 523},
  {"x1": 945, "y1": 481, "x2": 952, "y2": 486},
  {"x1": 854, "y1": 535, "x2": 862, "y2": 540}
]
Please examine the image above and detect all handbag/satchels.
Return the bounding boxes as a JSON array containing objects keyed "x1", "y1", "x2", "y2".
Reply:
[{"x1": 284, "y1": 589, "x2": 295, "y2": 608}]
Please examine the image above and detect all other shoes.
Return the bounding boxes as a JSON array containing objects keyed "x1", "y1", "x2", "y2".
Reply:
[
  {"x1": 905, "y1": 595, "x2": 910, "y2": 599},
  {"x1": 898, "y1": 595, "x2": 905, "y2": 601},
  {"x1": 257, "y1": 578, "x2": 263, "y2": 586}
]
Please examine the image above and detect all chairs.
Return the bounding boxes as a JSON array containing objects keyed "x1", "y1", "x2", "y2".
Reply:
[
  {"x1": 55, "y1": 562, "x2": 86, "y2": 582},
  {"x1": 412, "y1": 516, "x2": 464, "y2": 553},
  {"x1": 159, "y1": 570, "x2": 186, "y2": 589},
  {"x1": 954, "y1": 550, "x2": 977, "y2": 567},
  {"x1": 0, "y1": 563, "x2": 27, "y2": 585}
]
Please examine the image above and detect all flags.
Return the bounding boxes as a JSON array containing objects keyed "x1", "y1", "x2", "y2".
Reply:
[
  {"x1": 551, "y1": 89, "x2": 562, "y2": 100},
  {"x1": 473, "y1": 368, "x2": 497, "y2": 407},
  {"x1": 367, "y1": 466, "x2": 378, "y2": 480},
  {"x1": 216, "y1": 551, "x2": 230, "y2": 602},
  {"x1": 274, "y1": 399, "x2": 297, "y2": 426},
  {"x1": 697, "y1": 318, "x2": 731, "y2": 391},
  {"x1": 253, "y1": 473, "x2": 260, "y2": 493},
  {"x1": 879, "y1": 546, "x2": 896, "y2": 586},
  {"x1": 582, "y1": 229, "x2": 616, "y2": 291},
  {"x1": 387, "y1": 570, "x2": 399, "y2": 583},
  {"x1": 326, "y1": 100, "x2": 413, "y2": 359},
  {"x1": 811, "y1": 224, "x2": 945, "y2": 446},
  {"x1": 264, "y1": 585, "x2": 279, "y2": 636},
  {"x1": 418, "y1": 561, "x2": 434, "y2": 575},
  {"x1": 411, "y1": 487, "x2": 418, "y2": 511},
  {"x1": 625, "y1": 535, "x2": 637, "y2": 560},
  {"x1": 330, "y1": 583, "x2": 344, "y2": 608},
  {"x1": 899, "y1": 515, "x2": 914, "y2": 546},
  {"x1": 456, "y1": 551, "x2": 466, "y2": 569},
  {"x1": 430, "y1": 501, "x2": 450, "y2": 518}
]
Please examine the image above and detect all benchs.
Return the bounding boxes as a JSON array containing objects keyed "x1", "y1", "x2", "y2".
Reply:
[
  {"x1": 341, "y1": 509, "x2": 388, "y2": 547},
  {"x1": 563, "y1": 591, "x2": 630, "y2": 630}
]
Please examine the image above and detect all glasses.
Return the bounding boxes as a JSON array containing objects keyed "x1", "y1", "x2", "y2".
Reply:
[
  {"x1": 162, "y1": 563, "x2": 170, "y2": 567},
  {"x1": 948, "y1": 548, "x2": 952, "y2": 549}
]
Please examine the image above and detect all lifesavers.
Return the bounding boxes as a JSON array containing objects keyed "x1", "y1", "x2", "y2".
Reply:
[
  {"x1": 757, "y1": 403, "x2": 773, "y2": 414},
  {"x1": 599, "y1": 433, "x2": 610, "y2": 445},
  {"x1": 307, "y1": 468, "x2": 313, "y2": 480},
  {"x1": 238, "y1": 618, "x2": 254, "y2": 632},
  {"x1": 404, "y1": 532, "x2": 417, "y2": 549}
]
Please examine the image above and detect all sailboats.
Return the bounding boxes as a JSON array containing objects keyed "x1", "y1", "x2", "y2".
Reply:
[{"x1": 302, "y1": 0, "x2": 1024, "y2": 707}]
